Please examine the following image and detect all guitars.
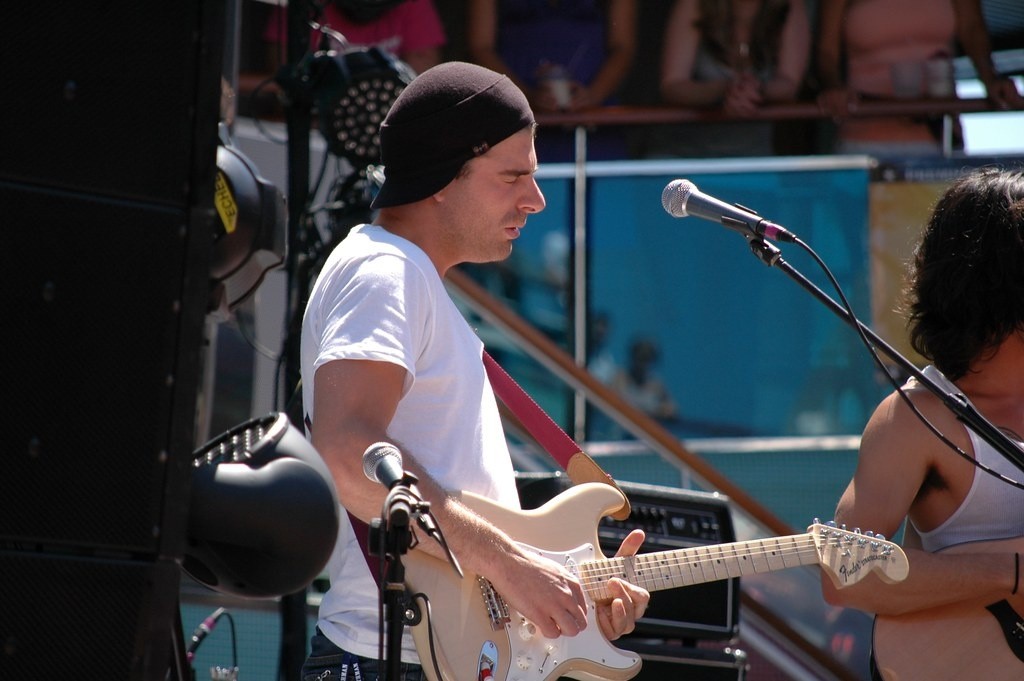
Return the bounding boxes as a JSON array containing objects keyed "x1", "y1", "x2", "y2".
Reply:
[
  {"x1": 872, "y1": 533, "x2": 1022, "y2": 681},
  {"x1": 393, "y1": 481, "x2": 912, "y2": 681}
]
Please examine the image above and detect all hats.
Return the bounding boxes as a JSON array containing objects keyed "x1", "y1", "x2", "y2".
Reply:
[{"x1": 370, "y1": 59, "x2": 534, "y2": 208}]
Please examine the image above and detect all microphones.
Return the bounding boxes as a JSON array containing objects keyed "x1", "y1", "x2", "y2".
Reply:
[
  {"x1": 662, "y1": 179, "x2": 797, "y2": 243},
  {"x1": 361, "y1": 443, "x2": 439, "y2": 535}
]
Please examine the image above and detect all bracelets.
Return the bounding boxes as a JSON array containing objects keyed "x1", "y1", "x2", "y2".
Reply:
[{"x1": 1011, "y1": 552, "x2": 1020, "y2": 595}]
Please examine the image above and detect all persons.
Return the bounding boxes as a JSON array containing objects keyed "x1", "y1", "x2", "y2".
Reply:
[
  {"x1": 301, "y1": 62, "x2": 649, "y2": 681},
  {"x1": 823, "y1": 167, "x2": 1024, "y2": 681},
  {"x1": 219, "y1": 0, "x2": 1020, "y2": 166}
]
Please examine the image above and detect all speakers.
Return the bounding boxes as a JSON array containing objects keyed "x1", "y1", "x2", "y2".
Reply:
[
  {"x1": 604, "y1": 640, "x2": 748, "y2": 681},
  {"x1": 0, "y1": 0, "x2": 232, "y2": 681}
]
